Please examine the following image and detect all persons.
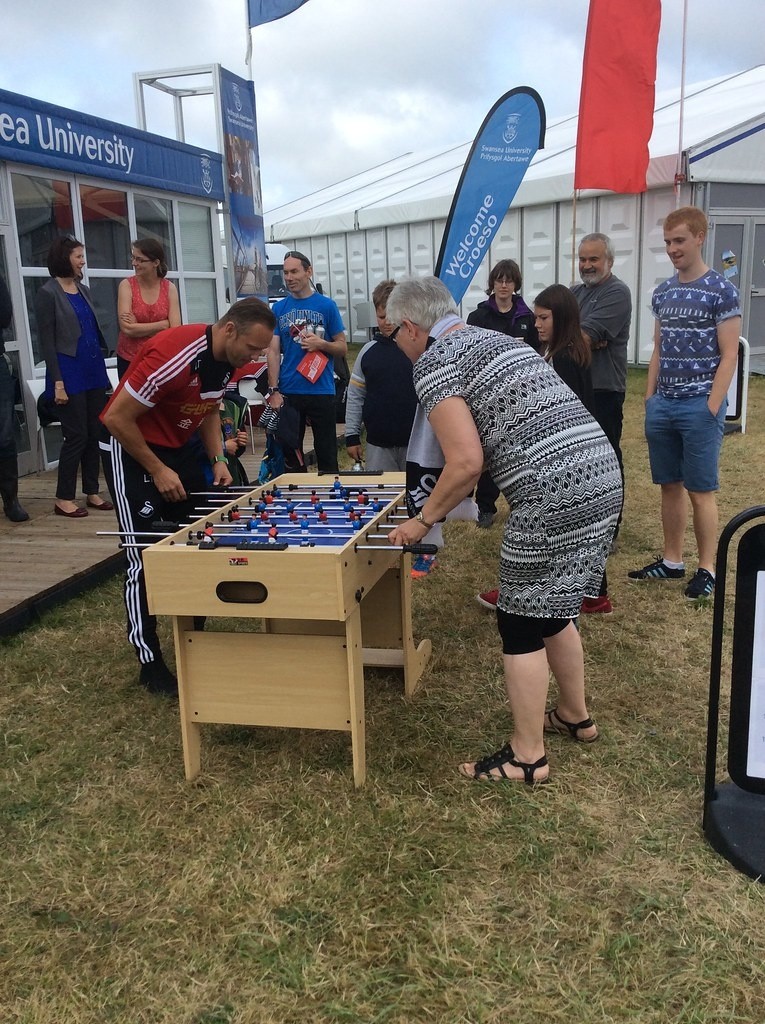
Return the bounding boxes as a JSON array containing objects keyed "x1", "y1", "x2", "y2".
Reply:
[
  {"x1": 203, "y1": 476, "x2": 383, "y2": 543},
  {"x1": 205, "y1": 389, "x2": 250, "y2": 492},
  {"x1": 267, "y1": 251, "x2": 348, "y2": 481},
  {"x1": 99, "y1": 297, "x2": 277, "y2": 691},
  {"x1": 0, "y1": 275, "x2": 31, "y2": 523},
  {"x1": 388, "y1": 276, "x2": 623, "y2": 785},
  {"x1": 466, "y1": 233, "x2": 634, "y2": 556},
  {"x1": 228, "y1": 152, "x2": 249, "y2": 195},
  {"x1": 345, "y1": 280, "x2": 442, "y2": 579},
  {"x1": 37, "y1": 235, "x2": 114, "y2": 517},
  {"x1": 117, "y1": 238, "x2": 181, "y2": 390},
  {"x1": 627, "y1": 207, "x2": 742, "y2": 600}
]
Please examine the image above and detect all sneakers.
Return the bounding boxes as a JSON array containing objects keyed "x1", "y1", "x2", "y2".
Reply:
[
  {"x1": 684, "y1": 567, "x2": 716, "y2": 602},
  {"x1": 410, "y1": 553, "x2": 438, "y2": 578},
  {"x1": 476, "y1": 513, "x2": 494, "y2": 528},
  {"x1": 628, "y1": 554, "x2": 685, "y2": 580},
  {"x1": 581, "y1": 594, "x2": 614, "y2": 615},
  {"x1": 138, "y1": 666, "x2": 179, "y2": 696},
  {"x1": 476, "y1": 589, "x2": 500, "y2": 609}
]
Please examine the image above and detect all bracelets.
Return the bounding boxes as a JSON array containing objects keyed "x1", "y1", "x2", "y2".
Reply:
[
  {"x1": 55, "y1": 387, "x2": 64, "y2": 390},
  {"x1": 269, "y1": 387, "x2": 279, "y2": 394},
  {"x1": 211, "y1": 456, "x2": 228, "y2": 466}
]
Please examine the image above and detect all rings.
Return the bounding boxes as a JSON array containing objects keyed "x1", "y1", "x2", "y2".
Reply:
[{"x1": 405, "y1": 542, "x2": 409, "y2": 546}]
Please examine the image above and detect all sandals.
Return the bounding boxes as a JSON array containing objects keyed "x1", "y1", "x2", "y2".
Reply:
[
  {"x1": 458, "y1": 743, "x2": 550, "y2": 785},
  {"x1": 541, "y1": 706, "x2": 600, "y2": 742}
]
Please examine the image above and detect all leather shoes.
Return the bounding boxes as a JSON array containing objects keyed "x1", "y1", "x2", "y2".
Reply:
[{"x1": 4, "y1": 502, "x2": 29, "y2": 523}]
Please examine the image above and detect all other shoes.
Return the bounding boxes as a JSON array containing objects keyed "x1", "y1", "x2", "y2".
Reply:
[
  {"x1": 86, "y1": 496, "x2": 113, "y2": 510},
  {"x1": 609, "y1": 539, "x2": 617, "y2": 555},
  {"x1": 55, "y1": 501, "x2": 89, "y2": 517}
]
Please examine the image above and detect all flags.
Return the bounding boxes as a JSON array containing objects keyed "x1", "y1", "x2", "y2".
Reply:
[
  {"x1": 247, "y1": 0, "x2": 310, "y2": 28},
  {"x1": 573, "y1": 0, "x2": 662, "y2": 193}
]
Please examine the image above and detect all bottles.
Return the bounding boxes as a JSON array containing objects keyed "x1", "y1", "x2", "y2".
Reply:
[
  {"x1": 307, "y1": 320, "x2": 314, "y2": 334},
  {"x1": 315, "y1": 320, "x2": 325, "y2": 339},
  {"x1": 352, "y1": 460, "x2": 364, "y2": 472}
]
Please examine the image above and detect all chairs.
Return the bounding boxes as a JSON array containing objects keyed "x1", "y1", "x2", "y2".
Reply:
[{"x1": 238, "y1": 375, "x2": 267, "y2": 455}]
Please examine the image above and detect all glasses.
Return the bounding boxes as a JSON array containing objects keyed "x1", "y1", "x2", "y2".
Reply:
[
  {"x1": 129, "y1": 255, "x2": 155, "y2": 263},
  {"x1": 494, "y1": 279, "x2": 517, "y2": 287},
  {"x1": 389, "y1": 324, "x2": 404, "y2": 343},
  {"x1": 61, "y1": 233, "x2": 75, "y2": 246},
  {"x1": 284, "y1": 253, "x2": 311, "y2": 266}
]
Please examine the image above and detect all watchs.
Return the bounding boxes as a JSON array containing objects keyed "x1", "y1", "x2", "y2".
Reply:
[{"x1": 416, "y1": 510, "x2": 435, "y2": 528}]
